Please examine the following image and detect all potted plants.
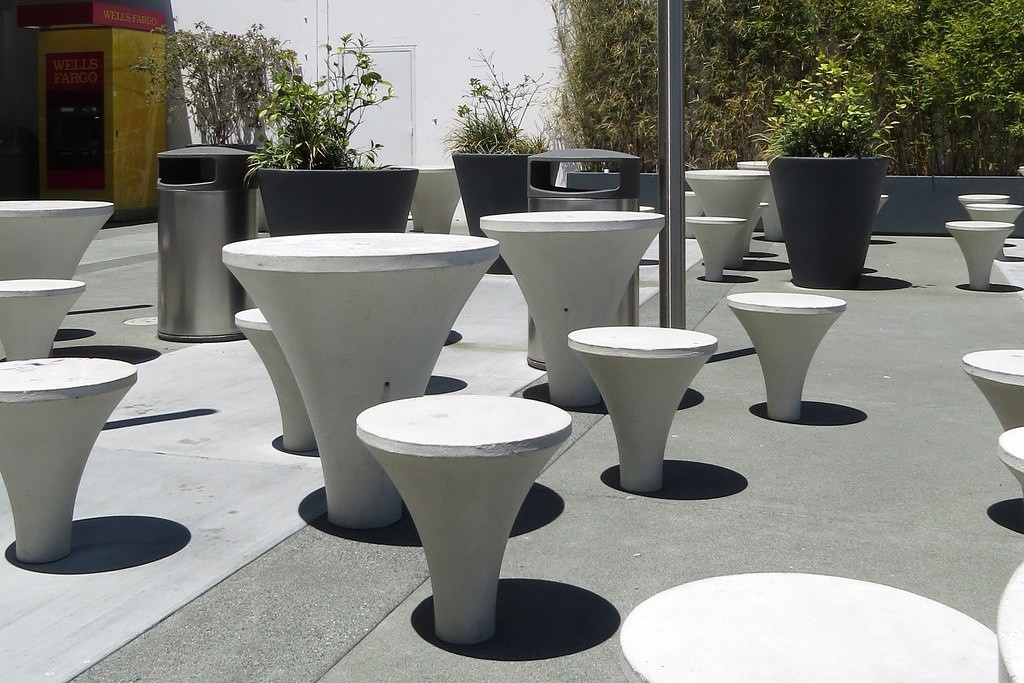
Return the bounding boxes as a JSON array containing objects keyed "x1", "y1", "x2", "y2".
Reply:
[
  {"x1": 241, "y1": 31, "x2": 420, "y2": 237},
  {"x1": 445, "y1": 46, "x2": 561, "y2": 273},
  {"x1": 749, "y1": 53, "x2": 909, "y2": 289}
]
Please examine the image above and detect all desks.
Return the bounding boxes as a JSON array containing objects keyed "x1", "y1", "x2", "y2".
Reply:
[
  {"x1": 736, "y1": 161, "x2": 784, "y2": 241},
  {"x1": 481, "y1": 210, "x2": 666, "y2": 404},
  {"x1": 225, "y1": 231, "x2": 499, "y2": 529},
  {"x1": 684, "y1": 169, "x2": 771, "y2": 257},
  {"x1": 0, "y1": 199, "x2": 116, "y2": 280}
]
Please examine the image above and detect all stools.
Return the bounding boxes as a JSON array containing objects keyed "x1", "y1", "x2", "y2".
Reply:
[
  {"x1": 640, "y1": 191, "x2": 770, "y2": 281},
  {"x1": 234, "y1": 306, "x2": 317, "y2": 451},
  {"x1": 353, "y1": 395, "x2": 574, "y2": 644},
  {"x1": 0, "y1": 357, "x2": 140, "y2": 565},
  {"x1": 0, "y1": 279, "x2": 87, "y2": 360},
  {"x1": 944, "y1": 194, "x2": 1024, "y2": 291},
  {"x1": 412, "y1": 168, "x2": 460, "y2": 233},
  {"x1": 567, "y1": 292, "x2": 1024, "y2": 683}
]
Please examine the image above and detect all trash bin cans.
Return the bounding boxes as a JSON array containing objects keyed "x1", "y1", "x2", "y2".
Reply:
[
  {"x1": 156, "y1": 145, "x2": 258, "y2": 343},
  {"x1": 525, "y1": 148, "x2": 641, "y2": 371}
]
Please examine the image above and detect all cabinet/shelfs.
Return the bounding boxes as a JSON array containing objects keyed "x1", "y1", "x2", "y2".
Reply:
[{"x1": 36, "y1": 25, "x2": 167, "y2": 221}]
